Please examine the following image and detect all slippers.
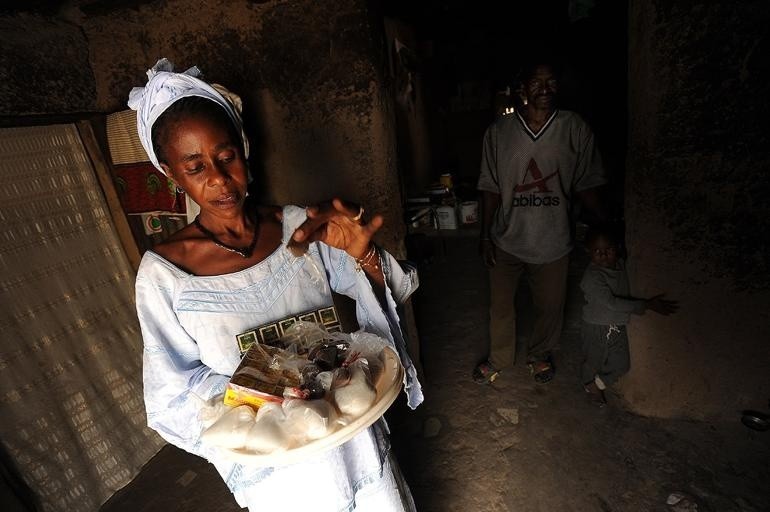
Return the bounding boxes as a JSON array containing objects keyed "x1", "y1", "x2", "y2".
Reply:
[
  {"x1": 471, "y1": 362, "x2": 503, "y2": 386},
  {"x1": 525, "y1": 352, "x2": 554, "y2": 384}
]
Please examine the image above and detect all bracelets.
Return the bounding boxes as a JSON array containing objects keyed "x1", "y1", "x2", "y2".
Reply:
[{"x1": 358, "y1": 242, "x2": 381, "y2": 272}]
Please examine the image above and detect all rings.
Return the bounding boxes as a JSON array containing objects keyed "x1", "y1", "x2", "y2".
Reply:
[{"x1": 353, "y1": 204, "x2": 365, "y2": 223}]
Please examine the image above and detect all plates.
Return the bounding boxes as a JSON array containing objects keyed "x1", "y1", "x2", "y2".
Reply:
[{"x1": 228, "y1": 344, "x2": 406, "y2": 459}]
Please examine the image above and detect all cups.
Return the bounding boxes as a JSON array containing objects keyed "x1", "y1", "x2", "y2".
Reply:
[{"x1": 460, "y1": 200, "x2": 479, "y2": 223}]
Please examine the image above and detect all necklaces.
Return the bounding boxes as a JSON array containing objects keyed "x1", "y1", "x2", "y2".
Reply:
[{"x1": 194, "y1": 204, "x2": 260, "y2": 259}]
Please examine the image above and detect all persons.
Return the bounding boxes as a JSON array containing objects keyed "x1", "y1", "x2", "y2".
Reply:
[
  {"x1": 578, "y1": 235, "x2": 680, "y2": 407},
  {"x1": 123, "y1": 55, "x2": 425, "y2": 512},
  {"x1": 472, "y1": 63, "x2": 589, "y2": 386}
]
